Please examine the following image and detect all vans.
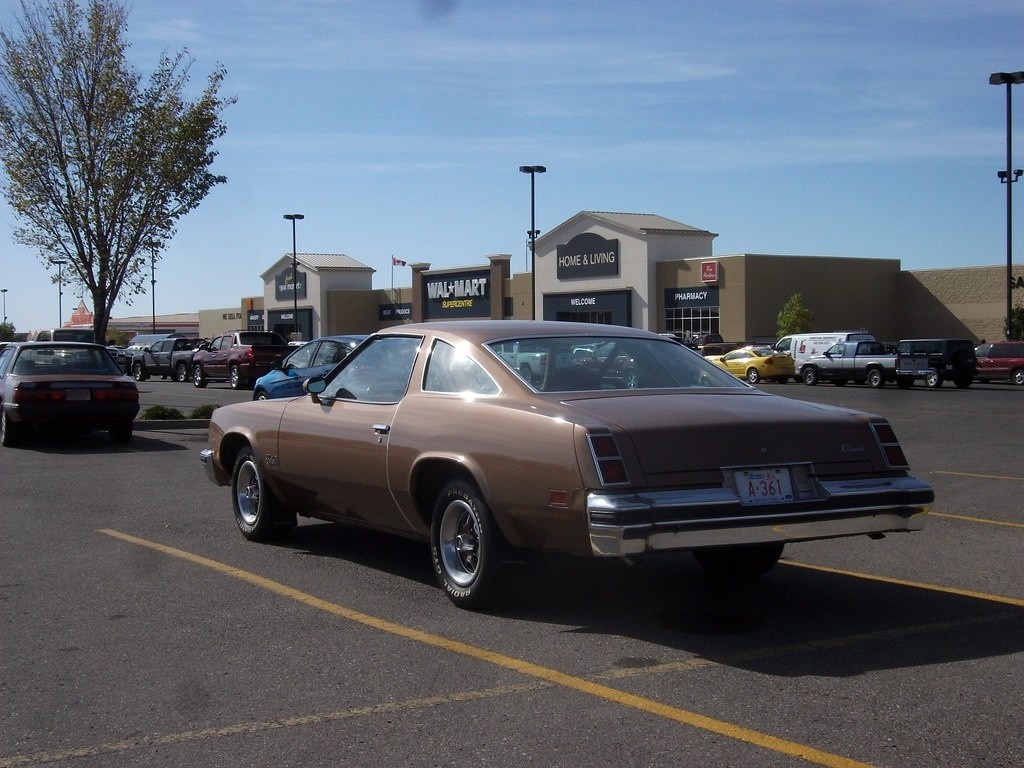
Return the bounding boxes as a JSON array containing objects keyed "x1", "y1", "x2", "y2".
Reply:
[{"x1": 773, "y1": 332, "x2": 876, "y2": 384}]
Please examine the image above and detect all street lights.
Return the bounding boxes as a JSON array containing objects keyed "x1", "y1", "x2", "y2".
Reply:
[
  {"x1": 283, "y1": 213, "x2": 305, "y2": 331},
  {"x1": 253, "y1": 334, "x2": 385, "y2": 402},
  {"x1": 51, "y1": 260, "x2": 67, "y2": 327},
  {"x1": 0, "y1": 288, "x2": 8, "y2": 325},
  {"x1": 519, "y1": 164, "x2": 547, "y2": 320},
  {"x1": 988, "y1": 70, "x2": 1024, "y2": 341}
]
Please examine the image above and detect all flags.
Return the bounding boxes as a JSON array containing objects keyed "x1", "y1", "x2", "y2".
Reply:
[{"x1": 393, "y1": 258, "x2": 406, "y2": 266}]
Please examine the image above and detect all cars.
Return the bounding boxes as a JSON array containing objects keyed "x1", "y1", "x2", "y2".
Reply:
[
  {"x1": 0, "y1": 342, "x2": 139, "y2": 447},
  {"x1": 577, "y1": 342, "x2": 647, "y2": 390},
  {"x1": 107, "y1": 345, "x2": 155, "y2": 376},
  {"x1": 713, "y1": 347, "x2": 795, "y2": 386},
  {"x1": 567, "y1": 340, "x2": 604, "y2": 374}
]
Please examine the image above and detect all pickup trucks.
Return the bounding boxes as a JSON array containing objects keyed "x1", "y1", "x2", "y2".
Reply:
[
  {"x1": 132, "y1": 338, "x2": 213, "y2": 382},
  {"x1": 659, "y1": 333, "x2": 693, "y2": 349},
  {"x1": 493, "y1": 339, "x2": 574, "y2": 386},
  {"x1": 192, "y1": 330, "x2": 304, "y2": 388},
  {"x1": 795, "y1": 338, "x2": 935, "y2": 389},
  {"x1": 690, "y1": 334, "x2": 756, "y2": 357}
]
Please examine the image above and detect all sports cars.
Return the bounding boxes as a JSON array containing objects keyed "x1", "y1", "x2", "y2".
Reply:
[{"x1": 202, "y1": 321, "x2": 937, "y2": 610}]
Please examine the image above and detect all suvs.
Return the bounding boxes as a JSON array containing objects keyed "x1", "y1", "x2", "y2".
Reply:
[
  {"x1": 974, "y1": 341, "x2": 1024, "y2": 388},
  {"x1": 28, "y1": 328, "x2": 96, "y2": 366},
  {"x1": 889, "y1": 338, "x2": 978, "y2": 390}
]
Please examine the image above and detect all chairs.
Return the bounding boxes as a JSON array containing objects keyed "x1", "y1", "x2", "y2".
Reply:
[{"x1": 544, "y1": 363, "x2": 601, "y2": 392}]
